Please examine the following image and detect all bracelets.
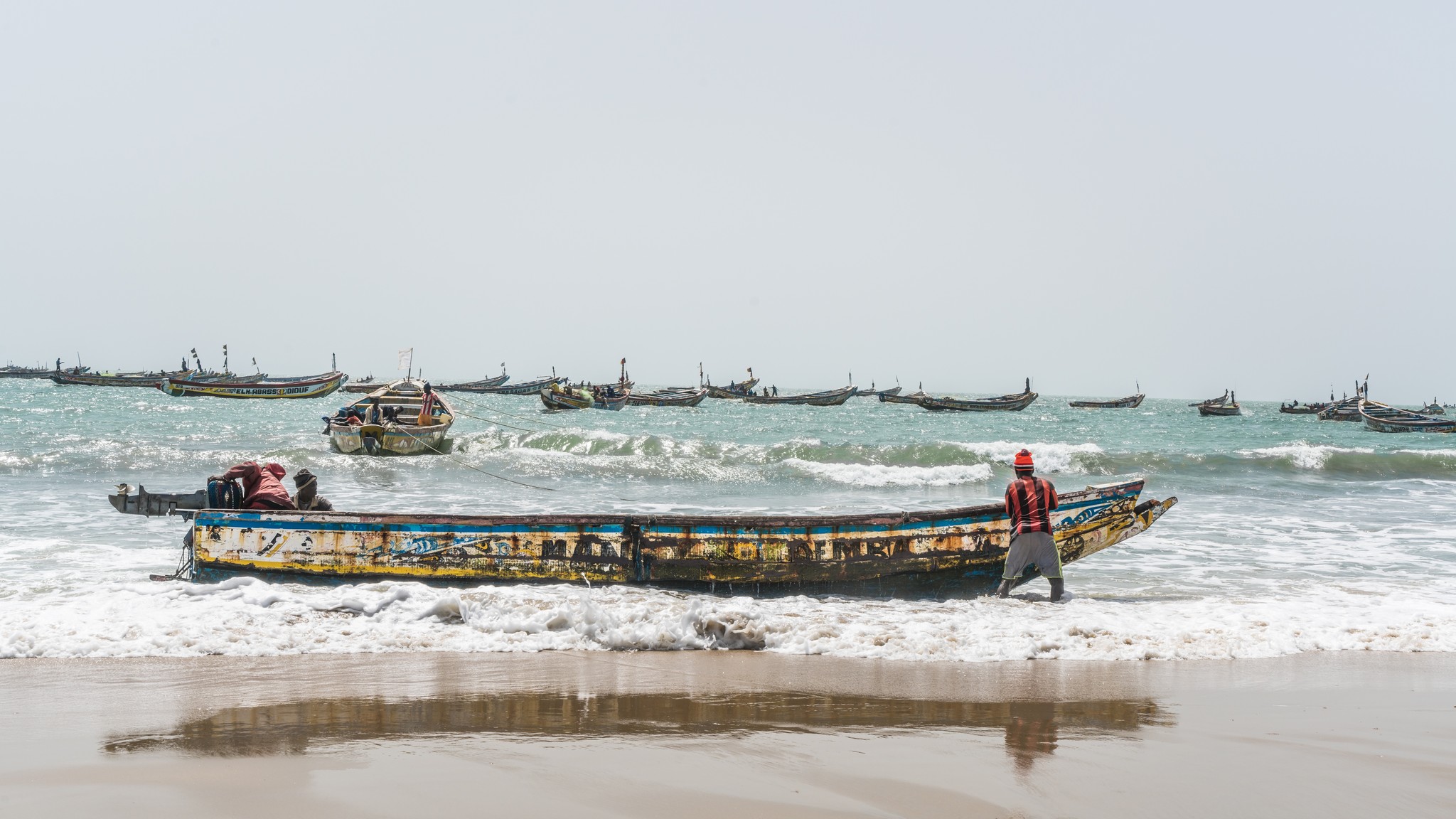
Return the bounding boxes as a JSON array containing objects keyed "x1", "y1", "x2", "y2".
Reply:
[
  {"x1": 222, "y1": 474, "x2": 225, "y2": 480},
  {"x1": 411, "y1": 423, "x2": 412, "y2": 425},
  {"x1": 446, "y1": 411, "x2": 448, "y2": 413}
]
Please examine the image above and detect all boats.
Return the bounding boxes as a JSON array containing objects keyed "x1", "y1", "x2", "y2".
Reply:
[
  {"x1": 540, "y1": 357, "x2": 635, "y2": 411},
  {"x1": 852, "y1": 375, "x2": 927, "y2": 404},
  {"x1": 1279, "y1": 373, "x2": 1456, "y2": 434},
  {"x1": 1188, "y1": 389, "x2": 1241, "y2": 416},
  {"x1": 916, "y1": 376, "x2": 1039, "y2": 411},
  {"x1": 697, "y1": 362, "x2": 858, "y2": 406},
  {"x1": 624, "y1": 386, "x2": 711, "y2": 407},
  {"x1": 321, "y1": 348, "x2": 567, "y2": 456},
  {"x1": 0, "y1": 352, "x2": 91, "y2": 379},
  {"x1": 49, "y1": 344, "x2": 349, "y2": 399},
  {"x1": 1069, "y1": 378, "x2": 1146, "y2": 409},
  {"x1": 100, "y1": 466, "x2": 1180, "y2": 587}
]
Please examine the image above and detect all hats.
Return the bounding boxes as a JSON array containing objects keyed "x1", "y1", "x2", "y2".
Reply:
[
  {"x1": 424, "y1": 382, "x2": 432, "y2": 390},
  {"x1": 387, "y1": 406, "x2": 394, "y2": 411},
  {"x1": 161, "y1": 370, "x2": 163, "y2": 371},
  {"x1": 1013, "y1": 448, "x2": 1035, "y2": 471},
  {"x1": 293, "y1": 469, "x2": 317, "y2": 491}
]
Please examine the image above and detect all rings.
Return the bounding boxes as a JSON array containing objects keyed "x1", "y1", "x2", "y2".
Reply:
[{"x1": 209, "y1": 477, "x2": 210, "y2": 479}]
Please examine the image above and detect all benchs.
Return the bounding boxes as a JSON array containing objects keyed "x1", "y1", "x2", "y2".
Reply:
[
  {"x1": 606, "y1": 391, "x2": 627, "y2": 400},
  {"x1": 356, "y1": 390, "x2": 443, "y2": 428},
  {"x1": 463, "y1": 376, "x2": 553, "y2": 389},
  {"x1": 586, "y1": 384, "x2": 622, "y2": 390},
  {"x1": 635, "y1": 390, "x2": 701, "y2": 401}
]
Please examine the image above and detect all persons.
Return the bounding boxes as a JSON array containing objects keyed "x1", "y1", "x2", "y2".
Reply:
[
  {"x1": 730, "y1": 380, "x2": 757, "y2": 396},
  {"x1": 56, "y1": 358, "x2": 165, "y2": 377},
  {"x1": 768, "y1": 385, "x2": 779, "y2": 397},
  {"x1": 547, "y1": 381, "x2": 559, "y2": 391},
  {"x1": 363, "y1": 397, "x2": 383, "y2": 426},
  {"x1": 1281, "y1": 400, "x2": 1325, "y2": 409},
  {"x1": 580, "y1": 380, "x2": 584, "y2": 387},
  {"x1": 30, "y1": 369, "x2": 32, "y2": 372},
  {"x1": 289, "y1": 469, "x2": 335, "y2": 512},
  {"x1": 563, "y1": 385, "x2": 572, "y2": 395},
  {"x1": 587, "y1": 381, "x2": 591, "y2": 387},
  {"x1": 996, "y1": 449, "x2": 1065, "y2": 601},
  {"x1": 705, "y1": 379, "x2": 710, "y2": 385},
  {"x1": 763, "y1": 386, "x2": 770, "y2": 396},
  {"x1": 380, "y1": 405, "x2": 404, "y2": 419},
  {"x1": 586, "y1": 385, "x2": 615, "y2": 408},
  {"x1": 339, "y1": 407, "x2": 366, "y2": 424},
  {"x1": 383, "y1": 406, "x2": 419, "y2": 426},
  {"x1": 565, "y1": 377, "x2": 576, "y2": 387},
  {"x1": 445, "y1": 384, "x2": 487, "y2": 388},
  {"x1": 417, "y1": 384, "x2": 452, "y2": 425},
  {"x1": 207, "y1": 461, "x2": 296, "y2": 510},
  {"x1": 327, "y1": 410, "x2": 364, "y2": 426}
]
